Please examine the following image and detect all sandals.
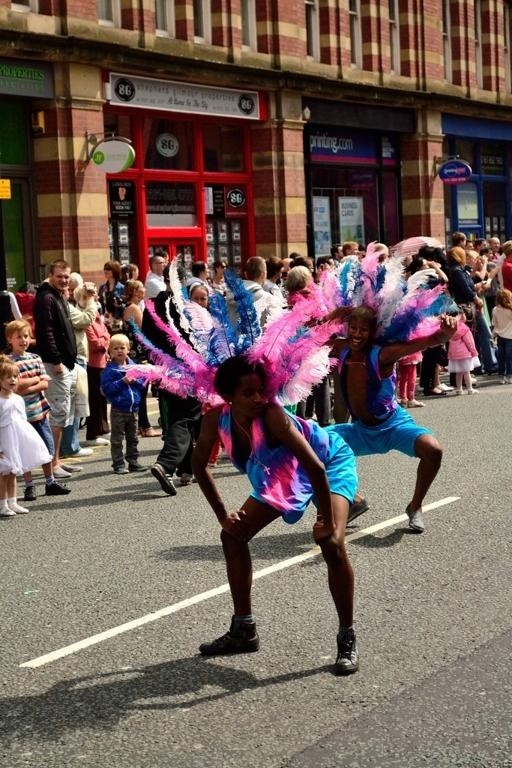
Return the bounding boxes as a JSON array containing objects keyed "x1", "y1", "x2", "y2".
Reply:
[
  {"x1": 456, "y1": 388, "x2": 480, "y2": 395},
  {"x1": 400, "y1": 398, "x2": 425, "y2": 409},
  {"x1": 499, "y1": 378, "x2": 512, "y2": 385},
  {"x1": 423, "y1": 384, "x2": 448, "y2": 396}
]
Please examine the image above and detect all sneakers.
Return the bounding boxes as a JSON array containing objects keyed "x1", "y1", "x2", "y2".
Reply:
[
  {"x1": 150, "y1": 463, "x2": 175, "y2": 496},
  {"x1": 336, "y1": 632, "x2": 360, "y2": 675},
  {"x1": 346, "y1": 498, "x2": 370, "y2": 524},
  {"x1": 219, "y1": 448, "x2": 230, "y2": 458},
  {"x1": 405, "y1": 501, "x2": 425, "y2": 532},
  {"x1": 199, "y1": 618, "x2": 260, "y2": 655},
  {"x1": 176, "y1": 472, "x2": 193, "y2": 485},
  {"x1": 0, "y1": 430, "x2": 162, "y2": 518}
]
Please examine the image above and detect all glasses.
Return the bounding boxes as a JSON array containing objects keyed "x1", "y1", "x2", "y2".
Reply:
[{"x1": 230, "y1": 387, "x2": 267, "y2": 400}]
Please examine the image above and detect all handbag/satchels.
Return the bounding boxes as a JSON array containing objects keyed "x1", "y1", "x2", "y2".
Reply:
[{"x1": 460, "y1": 302, "x2": 477, "y2": 325}]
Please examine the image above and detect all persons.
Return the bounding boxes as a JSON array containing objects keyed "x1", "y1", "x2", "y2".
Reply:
[
  {"x1": 0, "y1": 252, "x2": 316, "y2": 514},
  {"x1": 191, "y1": 354, "x2": 359, "y2": 676},
  {"x1": 318, "y1": 305, "x2": 461, "y2": 533},
  {"x1": 316, "y1": 231, "x2": 512, "y2": 425}
]
